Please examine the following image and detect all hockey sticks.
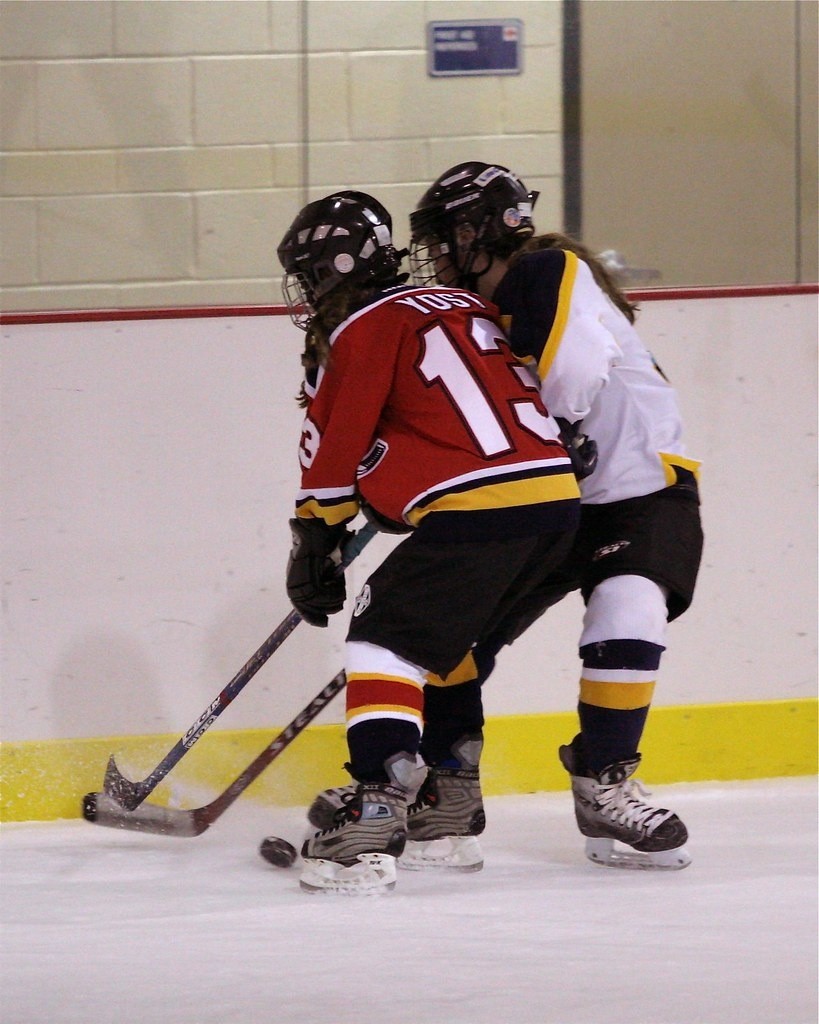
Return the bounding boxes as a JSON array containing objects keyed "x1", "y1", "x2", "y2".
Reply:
[
  {"x1": 102, "y1": 521, "x2": 378, "y2": 813},
  {"x1": 81, "y1": 667, "x2": 347, "y2": 838}
]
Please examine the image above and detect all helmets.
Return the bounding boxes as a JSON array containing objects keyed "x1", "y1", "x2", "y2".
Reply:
[
  {"x1": 409, "y1": 161, "x2": 535, "y2": 288},
  {"x1": 277, "y1": 190, "x2": 393, "y2": 331}
]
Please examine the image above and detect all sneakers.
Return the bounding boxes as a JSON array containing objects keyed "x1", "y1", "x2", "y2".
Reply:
[
  {"x1": 303, "y1": 785, "x2": 354, "y2": 840},
  {"x1": 560, "y1": 732, "x2": 692, "y2": 871},
  {"x1": 299, "y1": 752, "x2": 418, "y2": 894},
  {"x1": 395, "y1": 735, "x2": 486, "y2": 873}
]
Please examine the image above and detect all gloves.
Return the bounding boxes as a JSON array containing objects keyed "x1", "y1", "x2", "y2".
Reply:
[
  {"x1": 552, "y1": 419, "x2": 596, "y2": 477},
  {"x1": 285, "y1": 518, "x2": 356, "y2": 628}
]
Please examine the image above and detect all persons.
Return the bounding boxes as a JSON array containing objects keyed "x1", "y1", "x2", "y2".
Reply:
[
  {"x1": 274, "y1": 188, "x2": 581, "y2": 892},
  {"x1": 299, "y1": 162, "x2": 706, "y2": 870}
]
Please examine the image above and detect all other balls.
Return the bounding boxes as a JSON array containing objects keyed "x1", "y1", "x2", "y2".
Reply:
[{"x1": 257, "y1": 834, "x2": 299, "y2": 870}]
